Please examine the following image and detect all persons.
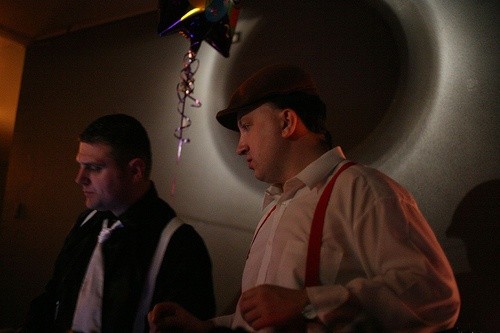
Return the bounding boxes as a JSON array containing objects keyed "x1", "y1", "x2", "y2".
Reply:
[
  {"x1": 148, "y1": 68, "x2": 460, "y2": 333},
  {"x1": 20, "y1": 113, "x2": 216, "y2": 333}
]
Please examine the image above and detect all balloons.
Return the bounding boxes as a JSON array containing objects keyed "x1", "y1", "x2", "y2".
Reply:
[{"x1": 158, "y1": 0, "x2": 240, "y2": 56}]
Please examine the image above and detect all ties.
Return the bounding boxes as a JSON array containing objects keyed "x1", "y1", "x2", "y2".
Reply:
[{"x1": 72, "y1": 220, "x2": 124, "y2": 333}]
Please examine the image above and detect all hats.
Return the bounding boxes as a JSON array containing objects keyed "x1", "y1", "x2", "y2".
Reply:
[{"x1": 216, "y1": 60, "x2": 313, "y2": 132}]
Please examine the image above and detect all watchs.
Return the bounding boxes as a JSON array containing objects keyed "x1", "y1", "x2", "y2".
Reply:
[{"x1": 301, "y1": 292, "x2": 318, "y2": 322}]
